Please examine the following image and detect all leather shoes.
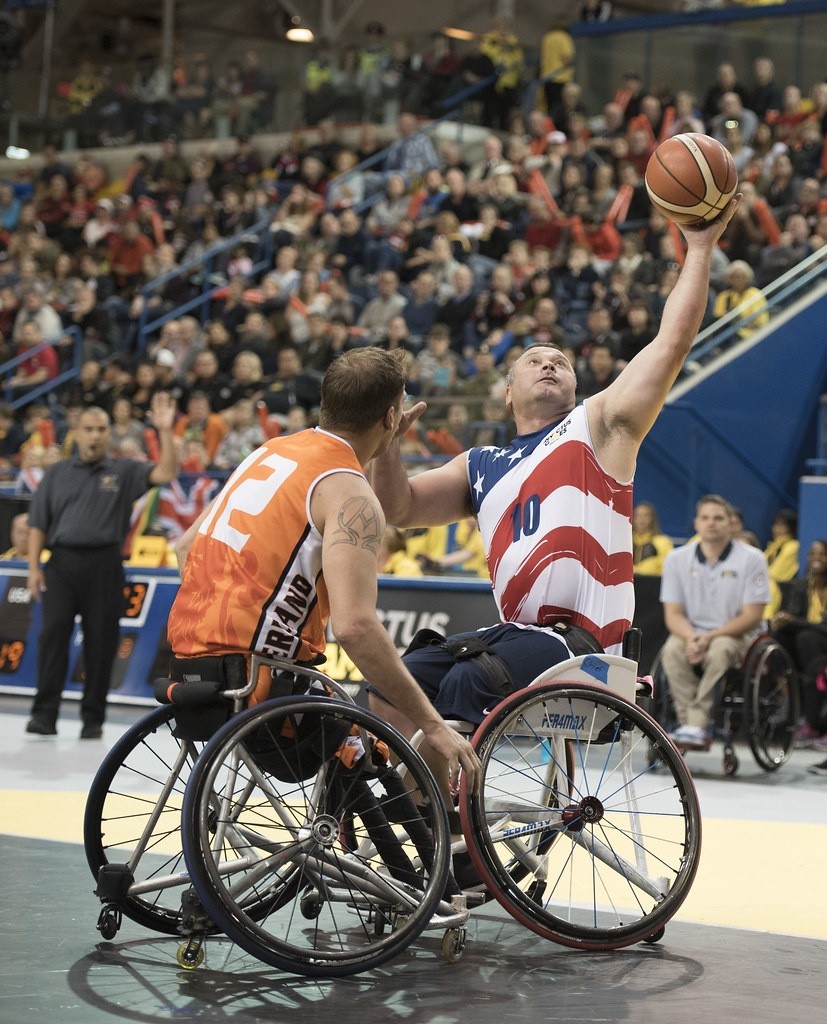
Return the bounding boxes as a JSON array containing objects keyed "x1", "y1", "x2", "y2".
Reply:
[
  {"x1": 25, "y1": 714, "x2": 57, "y2": 735},
  {"x1": 80, "y1": 723, "x2": 103, "y2": 739}
]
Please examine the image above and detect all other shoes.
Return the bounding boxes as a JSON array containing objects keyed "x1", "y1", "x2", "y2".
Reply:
[
  {"x1": 439, "y1": 842, "x2": 481, "y2": 886},
  {"x1": 806, "y1": 761, "x2": 827, "y2": 778}
]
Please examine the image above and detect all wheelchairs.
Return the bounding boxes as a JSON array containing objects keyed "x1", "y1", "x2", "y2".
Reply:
[
  {"x1": 647, "y1": 634, "x2": 796, "y2": 776},
  {"x1": 300, "y1": 627, "x2": 702, "y2": 962},
  {"x1": 83, "y1": 653, "x2": 470, "y2": 977}
]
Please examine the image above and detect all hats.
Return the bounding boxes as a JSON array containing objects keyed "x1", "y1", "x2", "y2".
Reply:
[{"x1": 156, "y1": 350, "x2": 177, "y2": 368}]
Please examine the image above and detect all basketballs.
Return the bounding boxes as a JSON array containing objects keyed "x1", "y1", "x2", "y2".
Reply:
[{"x1": 643, "y1": 130, "x2": 740, "y2": 228}]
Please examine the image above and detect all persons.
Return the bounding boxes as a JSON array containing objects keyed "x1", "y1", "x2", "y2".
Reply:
[
  {"x1": 25, "y1": 391, "x2": 178, "y2": 739},
  {"x1": 633, "y1": 493, "x2": 827, "y2": 776},
  {"x1": 365, "y1": 193, "x2": 742, "y2": 844},
  {"x1": 167, "y1": 346, "x2": 482, "y2": 797},
  {"x1": 0, "y1": 0, "x2": 827, "y2": 582}
]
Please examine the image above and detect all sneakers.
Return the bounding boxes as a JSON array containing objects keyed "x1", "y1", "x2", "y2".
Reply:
[{"x1": 666, "y1": 725, "x2": 713, "y2": 751}]
